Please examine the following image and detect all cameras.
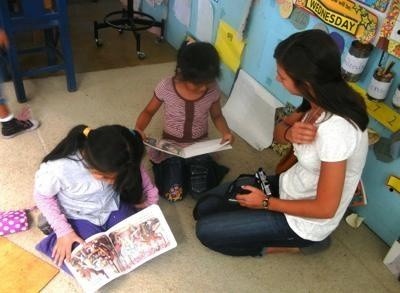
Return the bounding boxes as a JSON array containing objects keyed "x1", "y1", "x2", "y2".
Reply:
[{"x1": 227, "y1": 173, "x2": 260, "y2": 203}]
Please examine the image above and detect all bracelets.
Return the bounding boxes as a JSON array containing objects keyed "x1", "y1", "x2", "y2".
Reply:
[
  {"x1": 283, "y1": 124, "x2": 294, "y2": 143},
  {"x1": 263, "y1": 195, "x2": 271, "y2": 211}
]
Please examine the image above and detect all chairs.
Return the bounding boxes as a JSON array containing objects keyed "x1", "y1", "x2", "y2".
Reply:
[{"x1": 0, "y1": 0, "x2": 78, "y2": 103}]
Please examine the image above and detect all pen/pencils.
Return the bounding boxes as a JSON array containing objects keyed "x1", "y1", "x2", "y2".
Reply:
[{"x1": 367, "y1": 50, "x2": 396, "y2": 103}]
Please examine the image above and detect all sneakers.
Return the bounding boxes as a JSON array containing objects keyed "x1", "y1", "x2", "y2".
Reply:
[
  {"x1": 0, "y1": 117, "x2": 41, "y2": 139},
  {"x1": 36, "y1": 210, "x2": 54, "y2": 235},
  {"x1": 299, "y1": 234, "x2": 334, "y2": 256}
]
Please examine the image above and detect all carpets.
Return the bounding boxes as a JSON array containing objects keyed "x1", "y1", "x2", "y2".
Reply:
[{"x1": 0, "y1": 60, "x2": 400, "y2": 291}]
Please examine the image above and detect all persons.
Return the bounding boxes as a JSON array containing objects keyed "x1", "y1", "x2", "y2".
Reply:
[
  {"x1": 192, "y1": 27, "x2": 370, "y2": 257},
  {"x1": 0, "y1": 24, "x2": 42, "y2": 140},
  {"x1": 135, "y1": 35, "x2": 235, "y2": 203},
  {"x1": 32, "y1": 124, "x2": 159, "y2": 276}
]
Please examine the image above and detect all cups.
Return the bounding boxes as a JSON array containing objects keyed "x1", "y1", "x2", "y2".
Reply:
[
  {"x1": 364, "y1": 69, "x2": 394, "y2": 101},
  {"x1": 392, "y1": 84, "x2": 399, "y2": 109},
  {"x1": 341, "y1": 39, "x2": 373, "y2": 83}
]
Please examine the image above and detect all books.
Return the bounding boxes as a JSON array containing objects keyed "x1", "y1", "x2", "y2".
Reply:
[
  {"x1": 63, "y1": 203, "x2": 178, "y2": 292},
  {"x1": 142, "y1": 133, "x2": 232, "y2": 159}
]
